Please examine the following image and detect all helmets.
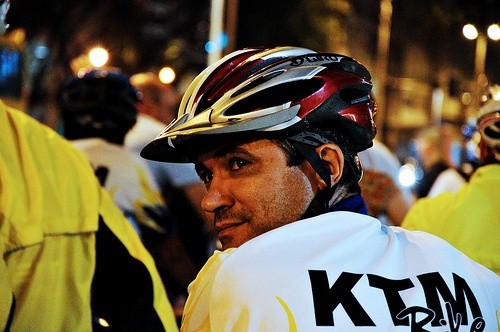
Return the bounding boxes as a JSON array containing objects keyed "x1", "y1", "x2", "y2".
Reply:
[
  {"x1": 139, "y1": 46, "x2": 375, "y2": 163},
  {"x1": 55, "y1": 70, "x2": 142, "y2": 145},
  {"x1": 477, "y1": 102, "x2": 500, "y2": 147}
]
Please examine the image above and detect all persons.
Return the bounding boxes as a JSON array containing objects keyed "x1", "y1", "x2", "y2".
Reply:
[
  {"x1": 141, "y1": 47, "x2": 500, "y2": 332},
  {"x1": 57, "y1": 68, "x2": 500, "y2": 310},
  {"x1": 0, "y1": 99, "x2": 178, "y2": 332}
]
